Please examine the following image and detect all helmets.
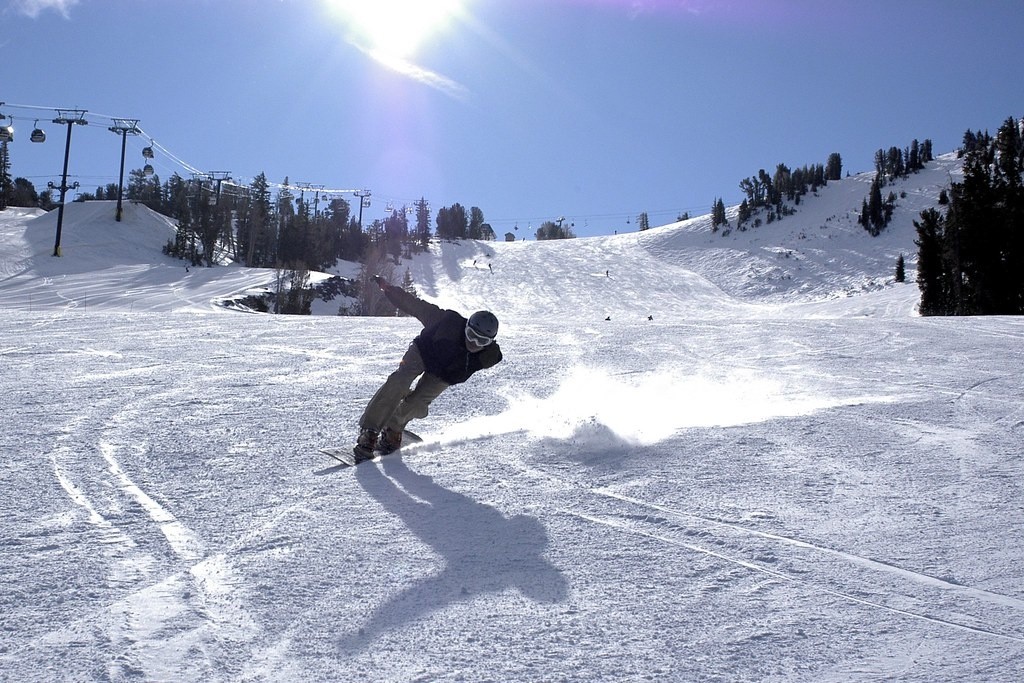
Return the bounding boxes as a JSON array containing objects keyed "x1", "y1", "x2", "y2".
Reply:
[{"x1": 467, "y1": 311, "x2": 499, "y2": 340}]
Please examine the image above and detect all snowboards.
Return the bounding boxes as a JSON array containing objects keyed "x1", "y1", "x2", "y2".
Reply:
[{"x1": 318, "y1": 429, "x2": 424, "y2": 467}]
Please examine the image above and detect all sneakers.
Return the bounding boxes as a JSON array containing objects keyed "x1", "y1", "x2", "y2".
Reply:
[
  {"x1": 357, "y1": 428, "x2": 378, "y2": 457},
  {"x1": 380, "y1": 428, "x2": 402, "y2": 453}
]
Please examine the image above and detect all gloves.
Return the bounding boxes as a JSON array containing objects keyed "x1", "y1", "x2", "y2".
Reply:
[{"x1": 370, "y1": 275, "x2": 389, "y2": 290}]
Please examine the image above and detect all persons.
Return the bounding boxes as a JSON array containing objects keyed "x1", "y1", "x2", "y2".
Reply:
[{"x1": 353, "y1": 273, "x2": 503, "y2": 465}]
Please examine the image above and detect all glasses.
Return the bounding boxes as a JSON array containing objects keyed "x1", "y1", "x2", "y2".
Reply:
[{"x1": 465, "y1": 324, "x2": 494, "y2": 347}]
